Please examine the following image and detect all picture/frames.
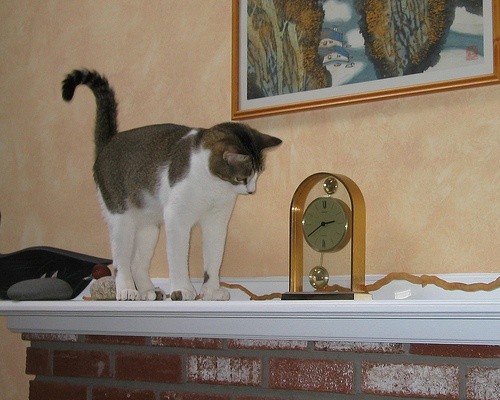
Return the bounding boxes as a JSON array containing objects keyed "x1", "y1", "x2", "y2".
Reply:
[{"x1": 231, "y1": 0, "x2": 500, "y2": 121}]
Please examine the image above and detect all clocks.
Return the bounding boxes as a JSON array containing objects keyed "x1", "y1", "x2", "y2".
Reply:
[{"x1": 280, "y1": 172, "x2": 372, "y2": 300}]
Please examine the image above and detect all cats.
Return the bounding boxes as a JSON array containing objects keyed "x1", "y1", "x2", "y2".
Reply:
[{"x1": 61, "y1": 67, "x2": 283, "y2": 300}]
